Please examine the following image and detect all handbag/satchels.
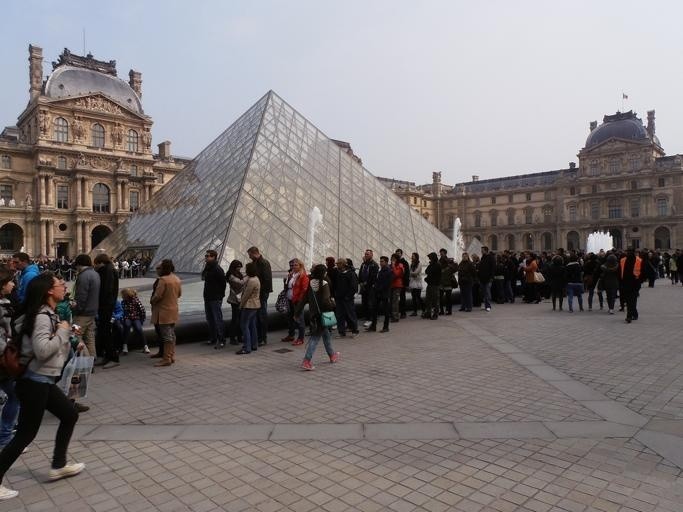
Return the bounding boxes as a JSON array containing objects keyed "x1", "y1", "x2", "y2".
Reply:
[
  {"x1": 0, "y1": 341, "x2": 26, "y2": 380},
  {"x1": 534, "y1": 272, "x2": 545, "y2": 282},
  {"x1": 276, "y1": 288, "x2": 290, "y2": 314}
]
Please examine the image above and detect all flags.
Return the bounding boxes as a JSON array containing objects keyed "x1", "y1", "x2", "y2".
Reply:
[{"x1": 623, "y1": 92, "x2": 628, "y2": 100}]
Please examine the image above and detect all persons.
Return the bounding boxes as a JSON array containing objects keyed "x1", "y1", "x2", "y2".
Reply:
[
  {"x1": 201, "y1": 246, "x2": 340, "y2": 370},
  {"x1": 650, "y1": 249, "x2": 682, "y2": 285},
  {"x1": 335, "y1": 248, "x2": 459, "y2": 340},
  {"x1": 93, "y1": 254, "x2": 182, "y2": 370},
  {"x1": 581, "y1": 245, "x2": 660, "y2": 323},
  {"x1": 1, "y1": 253, "x2": 100, "y2": 499},
  {"x1": 458, "y1": 245, "x2": 586, "y2": 313}
]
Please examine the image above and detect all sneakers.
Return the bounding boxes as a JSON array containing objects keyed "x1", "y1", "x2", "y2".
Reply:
[
  {"x1": 206, "y1": 307, "x2": 490, "y2": 370},
  {"x1": 76, "y1": 401, "x2": 89, "y2": 413},
  {"x1": 0, "y1": 485, "x2": 18, "y2": 501},
  {"x1": 50, "y1": 462, "x2": 85, "y2": 480},
  {"x1": 94, "y1": 339, "x2": 176, "y2": 369},
  {"x1": 569, "y1": 308, "x2": 638, "y2": 323}
]
